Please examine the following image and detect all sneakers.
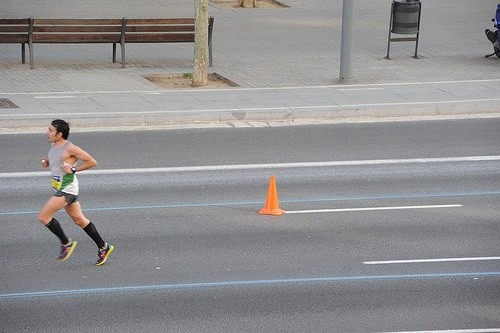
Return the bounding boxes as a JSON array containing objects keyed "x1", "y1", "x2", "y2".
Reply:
[
  {"x1": 94, "y1": 242, "x2": 114, "y2": 265},
  {"x1": 56, "y1": 238, "x2": 77, "y2": 263}
]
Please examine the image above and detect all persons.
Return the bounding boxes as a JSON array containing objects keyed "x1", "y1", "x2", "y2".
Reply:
[{"x1": 37, "y1": 119, "x2": 114, "y2": 267}]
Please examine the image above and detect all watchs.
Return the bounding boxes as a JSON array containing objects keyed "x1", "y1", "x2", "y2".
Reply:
[{"x1": 71, "y1": 166, "x2": 76, "y2": 174}]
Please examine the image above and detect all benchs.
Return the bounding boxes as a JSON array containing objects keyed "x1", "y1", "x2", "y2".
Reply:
[{"x1": 0, "y1": 16, "x2": 214, "y2": 70}]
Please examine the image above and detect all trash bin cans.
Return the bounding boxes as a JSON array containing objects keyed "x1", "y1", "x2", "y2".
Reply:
[{"x1": 391, "y1": 0, "x2": 420, "y2": 35}]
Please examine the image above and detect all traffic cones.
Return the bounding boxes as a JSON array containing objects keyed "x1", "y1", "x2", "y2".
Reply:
[{"x1": 259, "y1": 175, "x2": 285, "y2": 216}]
